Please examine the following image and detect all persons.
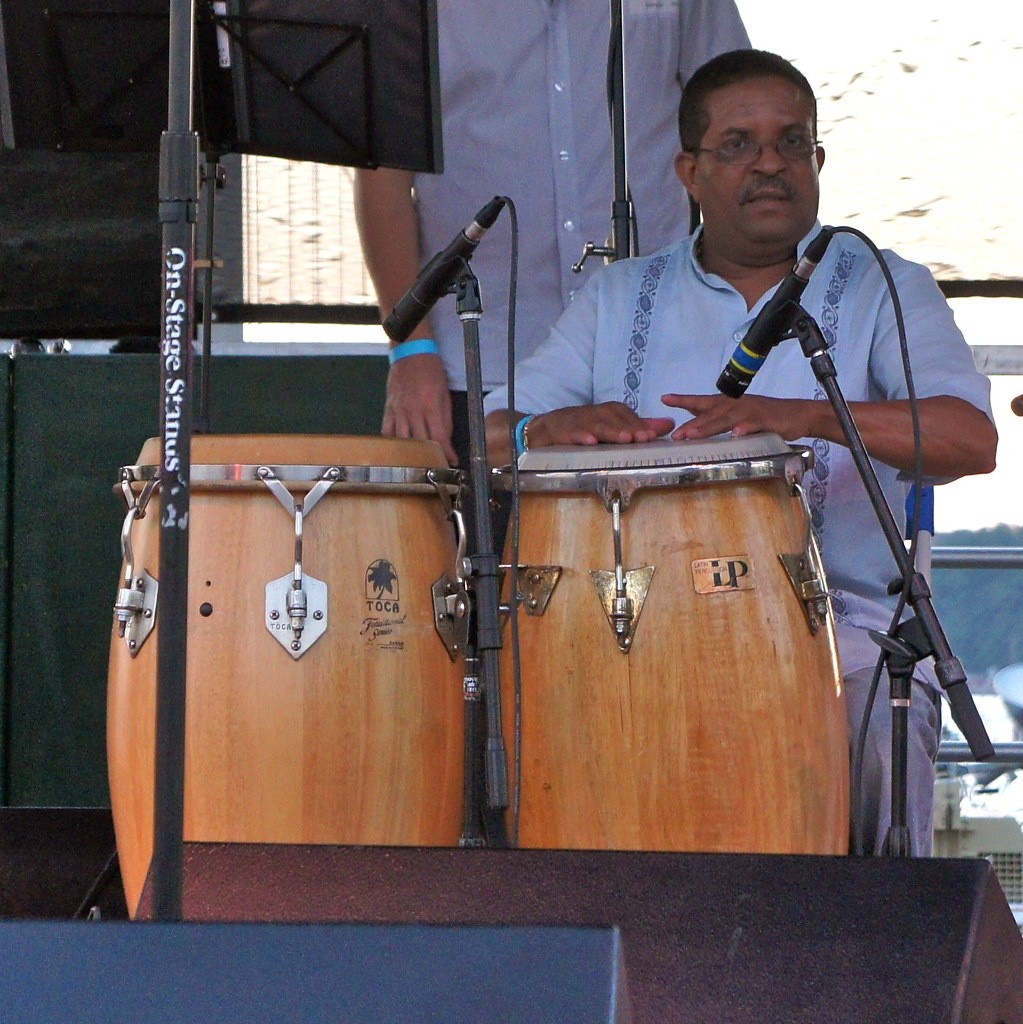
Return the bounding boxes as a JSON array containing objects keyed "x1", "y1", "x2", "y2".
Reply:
[
  {"x1": 354, "y1": 0, "x2": 752, "y2": 644},
  {"x1": 484, "y1": 47, "x2": 998, "y2": 858}
]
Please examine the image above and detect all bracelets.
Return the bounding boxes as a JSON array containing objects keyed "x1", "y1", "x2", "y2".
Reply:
[
  {"x1": 515, "y1": 414, "x2": 538, "y2": 458},
  {"x1": 388, "y1": 338, "x2": 437, "y2": 365}
]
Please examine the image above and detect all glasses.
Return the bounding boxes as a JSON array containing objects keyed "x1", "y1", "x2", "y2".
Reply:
[{"x1": 689, "y1": 132, "x2": 824, "y2": 166}]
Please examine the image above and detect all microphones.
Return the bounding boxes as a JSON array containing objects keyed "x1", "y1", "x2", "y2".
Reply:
[
  {"x1": 716, "y1": 228, "x2": 833, "y2": 398},
  {"x1": 382, "y1": 196, "x2": 505, "y2": 342}
]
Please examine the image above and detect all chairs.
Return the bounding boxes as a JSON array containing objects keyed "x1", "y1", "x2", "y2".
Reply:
[{"x1": 905, "y1": 482, "x2": 934, "y2": 588}]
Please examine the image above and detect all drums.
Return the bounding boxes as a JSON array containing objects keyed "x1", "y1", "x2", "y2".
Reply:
[
  {"x1": 103, "y1": 433, "x2": 475, "y2": 920},
  {"x1": 483, "y1": 427, "x2": 851, "y2": 857}
]
